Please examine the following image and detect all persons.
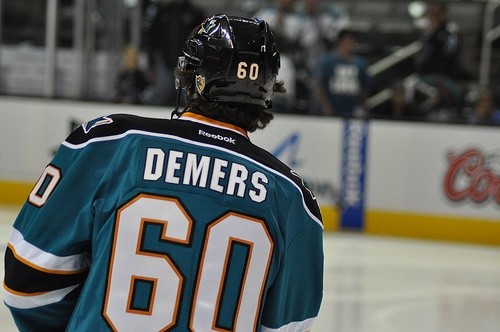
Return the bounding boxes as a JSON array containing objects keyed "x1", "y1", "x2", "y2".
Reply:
[
  {"x1": 0, "y1": 3, "x2": 498, "y2": 130},
  {"x1": 1, "y1": 15, "x2": 325, "y2": 332}
]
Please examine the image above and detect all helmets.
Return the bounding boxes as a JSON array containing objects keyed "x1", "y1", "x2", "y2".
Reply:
[{"x1": 173, "y1": 12, "x2": 279, "y2": 116}]
